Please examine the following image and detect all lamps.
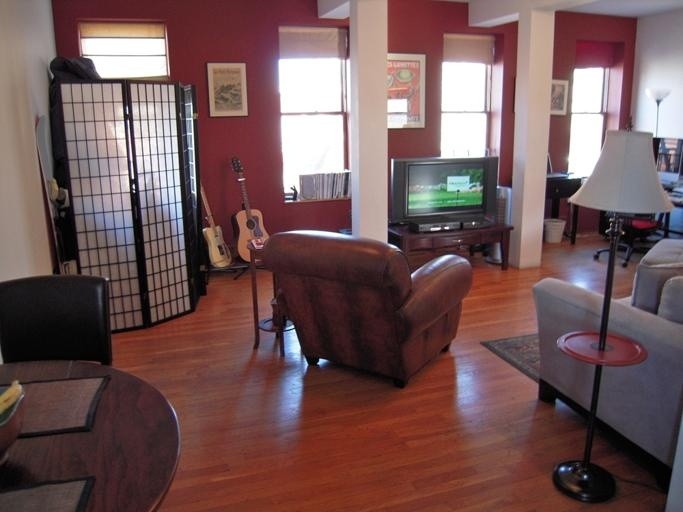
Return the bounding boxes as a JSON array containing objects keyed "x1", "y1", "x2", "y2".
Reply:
[
  {"x1": 646, "y1": 88, "x2": 670, "y2": 138},
  {"x1": 552, "y1": 130, "x2": 674, "y2": 503}
]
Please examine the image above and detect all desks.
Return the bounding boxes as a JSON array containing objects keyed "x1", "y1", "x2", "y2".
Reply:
[{"x1": 544, "y1": 172, "x2": 582, "y2": 245}]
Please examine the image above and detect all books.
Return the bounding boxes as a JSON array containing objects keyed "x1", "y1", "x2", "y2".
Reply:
[{"x1": 300, "y1": 171, "x2": 352, "y2": 200}]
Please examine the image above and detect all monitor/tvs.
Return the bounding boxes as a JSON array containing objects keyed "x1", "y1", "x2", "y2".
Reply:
[{"x1": 390, "y1": 156, "x2": 498, "y2": 224}]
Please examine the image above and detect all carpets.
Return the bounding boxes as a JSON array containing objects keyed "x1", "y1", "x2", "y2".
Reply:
[{"x1": 480, "y1": 332, "x2": 539, "y2": 383}]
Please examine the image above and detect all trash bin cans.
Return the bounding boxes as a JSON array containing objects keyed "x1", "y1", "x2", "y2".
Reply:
[{"x1": 544, "y1": 219, "x2": 566, "y2": 244}]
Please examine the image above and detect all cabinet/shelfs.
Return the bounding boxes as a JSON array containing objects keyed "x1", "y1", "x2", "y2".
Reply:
[{"x1": 388, "y1": 218, "x2": 514, "y2": 271}]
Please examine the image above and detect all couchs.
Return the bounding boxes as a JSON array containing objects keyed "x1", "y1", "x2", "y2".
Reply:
[
  {"x1": 260, "y1": 229, "x2": 473, "y2": 389},
  {"x1": 532, "y1": 239, "x2": 683, "y2": 469}
]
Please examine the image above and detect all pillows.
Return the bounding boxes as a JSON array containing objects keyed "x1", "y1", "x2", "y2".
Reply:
[{"x1": 630, "y1": 263, "x2": 683, "y2": 315}]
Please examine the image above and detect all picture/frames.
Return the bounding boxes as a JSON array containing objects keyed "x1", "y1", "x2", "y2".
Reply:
[
  {"x1": 206, "y1": 61, "x2": 248, "y2": 117},
  {"x1": 387, "y1": 52, "x2": 426, "y2": 130},
  {"x1": 550, "y1": 80, "x2": 569, "y2": 116}
]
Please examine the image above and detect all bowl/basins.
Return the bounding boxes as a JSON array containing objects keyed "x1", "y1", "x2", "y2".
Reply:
[{"x1": 0, "y1": 387, "x2": 25, "y2": 464}]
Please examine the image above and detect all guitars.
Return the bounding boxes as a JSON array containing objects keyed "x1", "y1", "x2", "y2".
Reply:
[
  {"x1": 201, "y1": 180, "x2": 232, "y2": 268},
  {"x1": 230, "y1": 157, "x2": 270, "y2": 262}
]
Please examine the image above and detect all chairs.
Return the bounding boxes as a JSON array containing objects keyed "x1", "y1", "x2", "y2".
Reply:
[
  {"x1": 0, "y1": 274, "x2": 112, "y2": 366},
  {"x1": 593, "y1": 187, "x2": 674, "y2": 268}
]
